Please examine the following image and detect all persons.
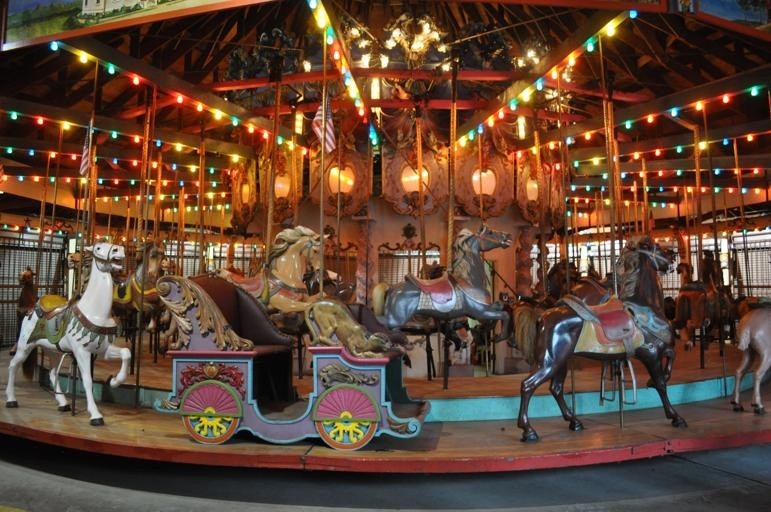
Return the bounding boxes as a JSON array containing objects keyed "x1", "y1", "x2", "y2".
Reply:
[{"x1": 702, "y1": 248, "x2": 722, "y2": 284}]
